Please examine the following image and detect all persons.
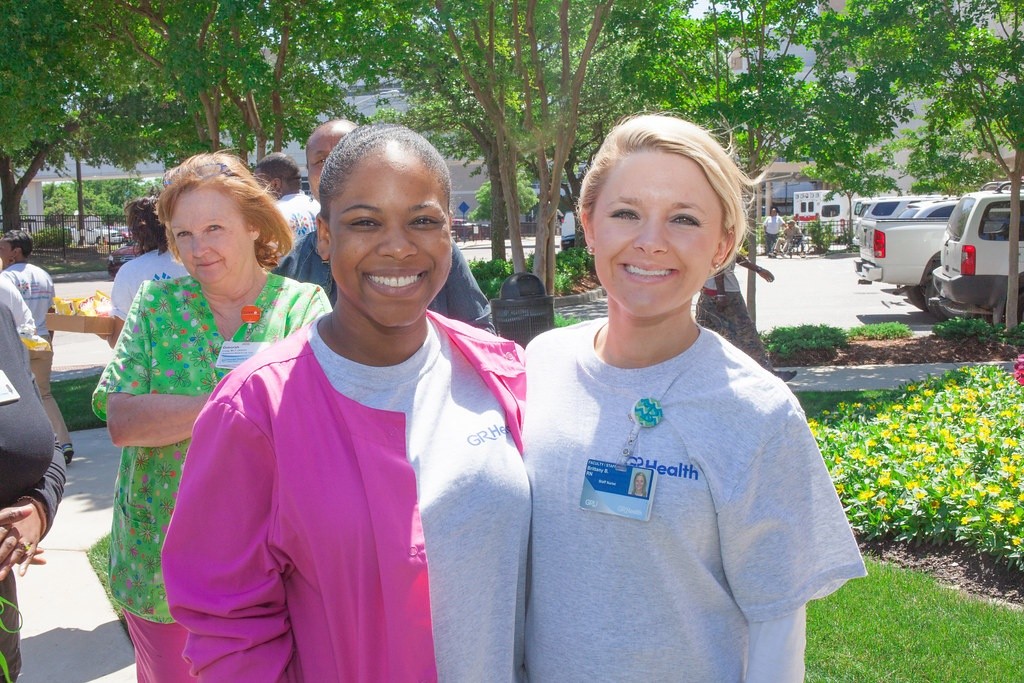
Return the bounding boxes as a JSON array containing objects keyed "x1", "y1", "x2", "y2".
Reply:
[
  {"x1": 763, "y1": 208, "x2": 785, "y2": 258},
  {"x1": 0, "y1": 229, "x2": 75, "y2": 683},
  {"x1": 161, "y1": 123, "x2": 533, "y2": 683},
  {"x1": 92, "y1": 154, "x2": 334, "y2": 683},
  {"x1": 631, "y1": 472, "x2": 648, "y2": 497},
  {"x1": 254, "y1": 152, "x2": 321, "y2": 267},
  {"x1": 524, "y1": 115, "x2": 866, "y2": 683},
  {"x1": 696, "y1": 251, "x2": 797, "y2": 383},
  {"x1": 769, "y1": 220, "x2": 803, "y2": 257},
  {"x1": 96, "y1": 196, "x2": 190, "y2": 349},
  {"x1": 271, "y1": 119, "x2": 496, "y2": 335}
]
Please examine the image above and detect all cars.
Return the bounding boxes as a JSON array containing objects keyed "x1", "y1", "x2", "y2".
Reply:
[
  {"x1": 107, "y1": 238, "x2": 147, "y2": 276},
  {"x1": 858, "y1": 198, "x2": 957, "y2": 289},
  {"x1": 74, "y1": 226, "x2": 123, "y2": 243}
]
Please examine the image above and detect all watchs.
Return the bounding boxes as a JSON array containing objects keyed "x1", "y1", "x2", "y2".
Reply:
[{"x1": 756, "y1": 266, "x2": 762, "y2": 273}]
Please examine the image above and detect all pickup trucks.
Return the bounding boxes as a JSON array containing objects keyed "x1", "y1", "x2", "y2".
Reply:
[{"x1": 855, "y1": 217, "x2": 1009, "y2": 314}]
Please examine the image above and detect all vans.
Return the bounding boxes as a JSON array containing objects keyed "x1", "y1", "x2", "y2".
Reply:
[{"x1": 928, "y1": 182, "x2": 1024, "y2": 327}]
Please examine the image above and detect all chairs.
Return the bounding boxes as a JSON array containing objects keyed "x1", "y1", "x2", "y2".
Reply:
[{"x1": 453, "y1": 221, "x2": 490, "y2": 241}]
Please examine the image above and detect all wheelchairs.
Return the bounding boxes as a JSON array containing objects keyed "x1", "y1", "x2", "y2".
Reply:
[{"x1": 782, "y1": 234, "x2": 806, "y2": 259}]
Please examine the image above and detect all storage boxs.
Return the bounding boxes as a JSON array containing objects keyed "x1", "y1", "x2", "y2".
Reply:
[
  {"x1": 28, "y1": 335, "x2": 54, "y2": 378},
  {"x1": 45, "y1": 313, "x2": 115, "y2": 336}
]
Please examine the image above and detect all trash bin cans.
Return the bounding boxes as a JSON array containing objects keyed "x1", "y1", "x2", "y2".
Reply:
[{"x1": 488, "y1": 267, "x2": 559, "y2": 349}]
